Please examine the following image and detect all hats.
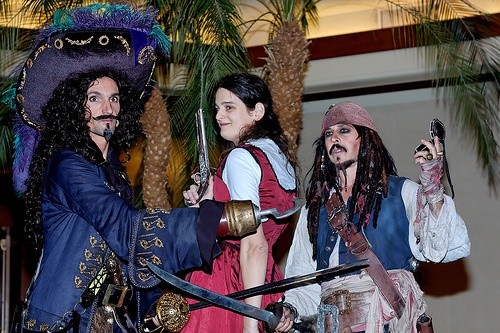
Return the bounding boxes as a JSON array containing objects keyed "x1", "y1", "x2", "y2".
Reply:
[
  {"x1": 17, "y1": 28, "x2": 157, "y2": 131},
  {"x1": 322, "y1": 103, "x2": 377, "y2": 133}
]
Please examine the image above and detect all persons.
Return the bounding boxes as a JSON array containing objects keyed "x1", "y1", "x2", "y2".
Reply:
[
  {"x1": 21, "y1": 68, "x2": 260, "y2": 333},
  {"x1": 274, "y1": 100, "x2": 472, "y2": 333},
  {"x1": 140, "y1": 72, "x2": 298, "y2": 333}
]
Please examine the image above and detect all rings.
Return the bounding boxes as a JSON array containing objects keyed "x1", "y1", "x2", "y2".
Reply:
[
  {"x1": 437, "y1": 151, "x2": 444, "y2": 156},
  {"x1": 426, "y1": 154, "x2": 433, "y2": 160}
]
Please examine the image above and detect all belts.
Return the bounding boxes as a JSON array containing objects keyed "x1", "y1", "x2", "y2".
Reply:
[
  {"x1": 81, "y1": 283, "x2": 137, "y2": 309},
  {"x1": 301, "y1": 289, "x2": 376, "y2": 333}
]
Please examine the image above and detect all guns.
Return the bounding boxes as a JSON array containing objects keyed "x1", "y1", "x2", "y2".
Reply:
[{"x1": 183, "y1": 108, "x2": 211, "y2": 205}]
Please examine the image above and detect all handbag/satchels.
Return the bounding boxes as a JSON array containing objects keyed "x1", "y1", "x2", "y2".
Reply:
[{"x1": 416, "y1": 314, "x2": 434, "y2": 333}]
